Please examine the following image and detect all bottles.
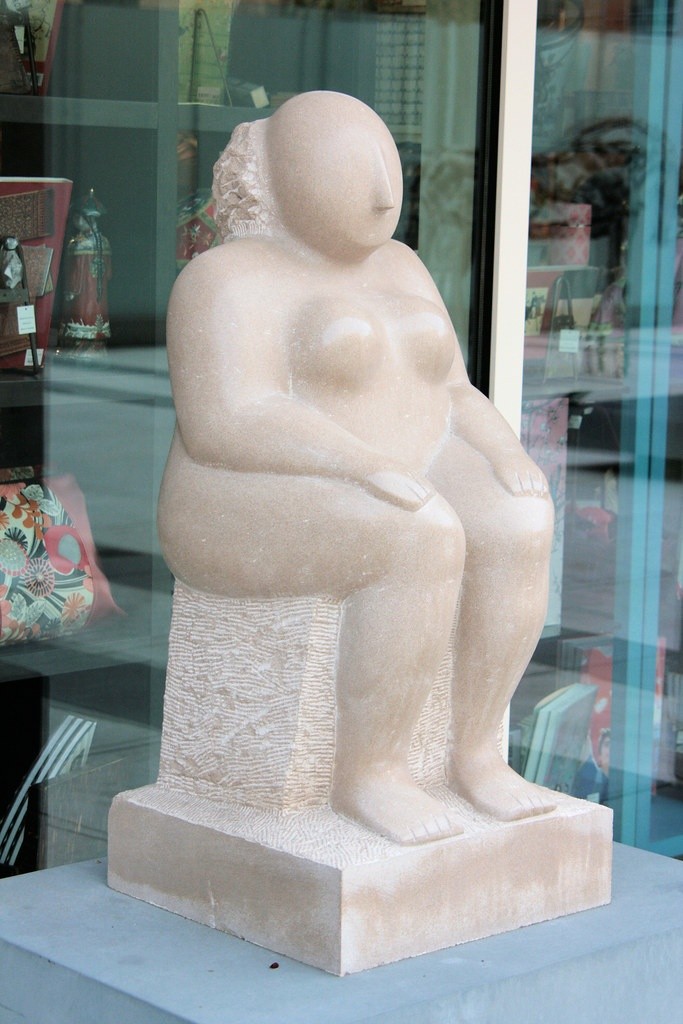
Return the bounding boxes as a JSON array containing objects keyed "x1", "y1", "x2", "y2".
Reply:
[{"x1": 59, "y1": 188, "x2": 111, "y2": 342}]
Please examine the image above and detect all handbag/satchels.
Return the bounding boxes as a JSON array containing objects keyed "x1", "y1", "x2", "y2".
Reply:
[{"x1": 0, "y1": 474, "x2": 130, "y2": 651}]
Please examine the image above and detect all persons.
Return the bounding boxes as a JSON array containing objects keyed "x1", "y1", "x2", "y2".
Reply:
[
  {"x1": 156, "y1": 90, "x2": 556, "y2": 845},
  {"x1": 59, "y1": 196, "x2": 112, "y2": 352}
]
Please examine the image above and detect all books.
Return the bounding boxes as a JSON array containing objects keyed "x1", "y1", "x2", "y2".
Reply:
[{"x1": 0, "y1": 177, "x2": 73, "y2": 370}]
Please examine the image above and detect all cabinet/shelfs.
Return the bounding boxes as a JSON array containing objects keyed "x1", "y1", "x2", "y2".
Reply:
[{"x1": 0, "y1": 0, "x2": 277, "y2": 684}]
